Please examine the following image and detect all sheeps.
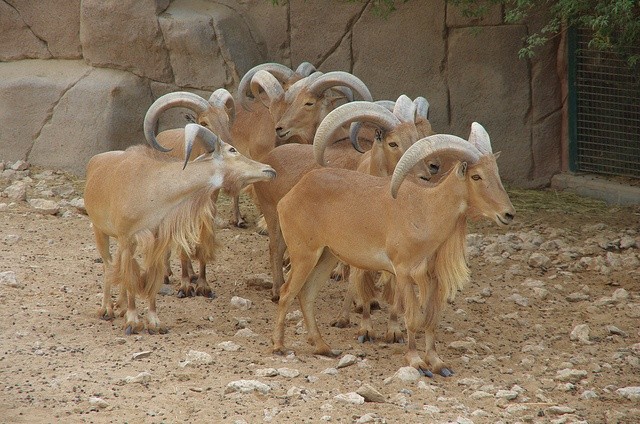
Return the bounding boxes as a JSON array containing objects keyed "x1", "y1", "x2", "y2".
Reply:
[
  {"x1": 324, "y1": 96, "x2": 439, "y2": 328},
  {"x1": 272, "y1": 122, "x2": 517, "y2": 377},
  {"x1": 225, "y1": 62, "x2": 317, "y2": 233},
  {"x1": 275, "y1": 71, "x2": 374, "y2": 282},
  {"x1": 249, "y1": 70, "x2": 324, "y2": 274},
  {"x1": 127, "y1": 87, "x2": 235, "y2": 299},
  {"x1": 85, "y1": 125, "x2": 278, "y2": 336},
  {"x1": 254, "y1": 94, "x2": 431, "y2": 346}
]
